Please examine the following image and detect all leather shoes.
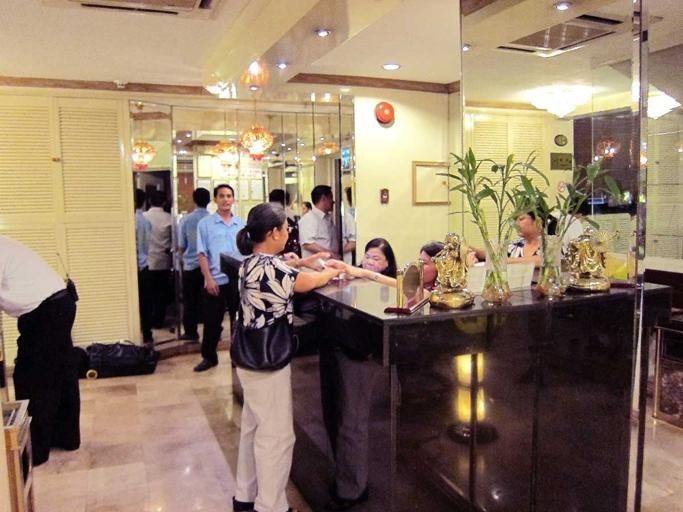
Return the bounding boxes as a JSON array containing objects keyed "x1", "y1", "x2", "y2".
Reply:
[
  {"x1": 181, "y1": 332, "x2": 200, "y2": 340},
  {"x1": 194, "y1": 356, "x2": 218, "y2": 371}
]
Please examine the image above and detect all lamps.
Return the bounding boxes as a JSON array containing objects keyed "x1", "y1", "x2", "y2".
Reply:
[
  {"x1": 131, "y1": 110, "x2": 157, "y2": 171},
  {"x1": 240, "y1": 86, "x2": 274, "y2": 162},
  {"x1": 446, "y1": 353, "x2": 498, "y2": 445},
  {"x1": 209, "y1": 101, "x2": 241, "y2": 168}
]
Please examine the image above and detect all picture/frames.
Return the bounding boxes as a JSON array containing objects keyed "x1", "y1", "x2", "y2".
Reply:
[{"x1": 411, "y1": 161, "x2": 453, "y2": 206}]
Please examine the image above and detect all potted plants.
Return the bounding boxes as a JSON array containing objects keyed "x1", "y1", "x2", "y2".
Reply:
[
  {"x1": 527, "y1": 153, "x2": 624, "y2": 303},
  {"x1": 448, "y1": 147, "x2": 549, "y2": 301}
]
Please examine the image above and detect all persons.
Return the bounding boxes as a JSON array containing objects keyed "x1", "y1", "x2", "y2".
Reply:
[
  {"x1": 134, "y1": 189, "x2": 151, "y2": 343},
  {"x1": 355, "y1": 238, "x2": 398, "y2": 278},
  {"x1": 142, "y1": 191, "x2": 171, "y2": 328},
  {"x1": 0, "y1": 233, "x2": 80, "y2": 467},
  {"x1": 228, "y1": 202, "x2": 346, "y2": 511},
  {"x1": 267, "y1": 189, "x2": 301, "y2": 228},
  {"x1": 296, "y1": 185, "x2": 342, "y2": 259},
  {"x1": 298, "y1": 202, "x2": 311, "y2": 214},
  {"x1": 558, "y1": 198, "x2": 590, "y2": 280},
  {"x1": 325, "y1": 240, "x2": 447, "y2": 292},
  {"x1": 177, "y1": 188, "x2": 211, "y2": 341},
  {"x1": 192, "y1": 185, "x2": 247, "y2": 372},
  {"x1": 468, "y1": 196, "x2": 558, "y2": 286}
]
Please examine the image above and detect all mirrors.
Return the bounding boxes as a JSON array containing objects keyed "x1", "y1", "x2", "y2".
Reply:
[
  {"x1": 624, "y1": 2, "x2": 682, "y2": 512},
  {"x1": 171, "y1": 92, "x2": 355, "y2": 346},
  {"x1": 459, "y1": 1, "x2": 645, "y2": 292},
  {"x1": 129, "y1": 100, "x2": 182, "y2": 346}
]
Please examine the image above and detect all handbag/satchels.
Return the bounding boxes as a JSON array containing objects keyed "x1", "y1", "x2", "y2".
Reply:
[
  {"x1": 230, "y1": 317, "x2": 295, "y2": 372},
  {"x1": 88, "y1": 342, "x2": 160, "y2": 378}
]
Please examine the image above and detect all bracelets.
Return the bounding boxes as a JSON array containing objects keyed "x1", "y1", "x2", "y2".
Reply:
[{"x1": 372, "y1": 271, "x2": 381, "y2": 281}]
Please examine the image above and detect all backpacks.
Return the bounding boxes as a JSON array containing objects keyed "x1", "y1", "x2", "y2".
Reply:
[{"x1": 281, "y1": 215, "x2": 301, "y2": 257}]
